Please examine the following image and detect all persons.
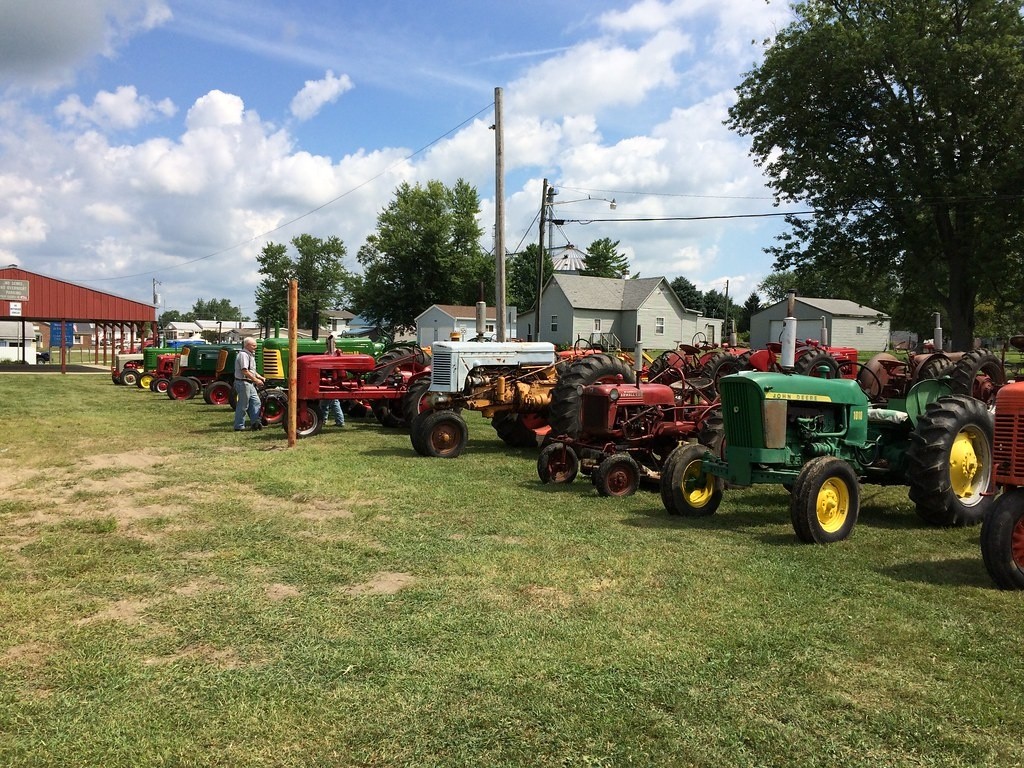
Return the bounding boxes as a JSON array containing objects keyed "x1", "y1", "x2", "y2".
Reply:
[
  {"x1": 319, "y1": 336, "x2": 345, "y2": 426},
  {"x1": 234, "y1": 337, "x2": 267, "y2": 431}
]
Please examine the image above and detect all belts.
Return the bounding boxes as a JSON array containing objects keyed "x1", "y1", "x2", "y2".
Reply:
[{"x1": 235, "y1": 378, "x2": 253, "y2": 384}]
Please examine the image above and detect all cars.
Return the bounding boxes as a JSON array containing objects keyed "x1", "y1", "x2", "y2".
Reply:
[{"x1": 36, "y1": 351, "x2": 50, "y2": 365}]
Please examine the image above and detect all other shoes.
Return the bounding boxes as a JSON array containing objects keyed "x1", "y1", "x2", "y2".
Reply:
[{"x1": 235, "y1": 427, "x2": 251, "y2": 432}]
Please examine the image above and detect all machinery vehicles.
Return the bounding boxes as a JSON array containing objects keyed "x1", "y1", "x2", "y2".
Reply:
[{"x1": 111, "y1": 285, "x2": 1024, "y2": 591}]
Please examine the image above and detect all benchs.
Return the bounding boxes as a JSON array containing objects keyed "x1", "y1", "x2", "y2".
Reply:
[{"x1": 866, "y1": 408, "x2": 909, "y2": 425}]
